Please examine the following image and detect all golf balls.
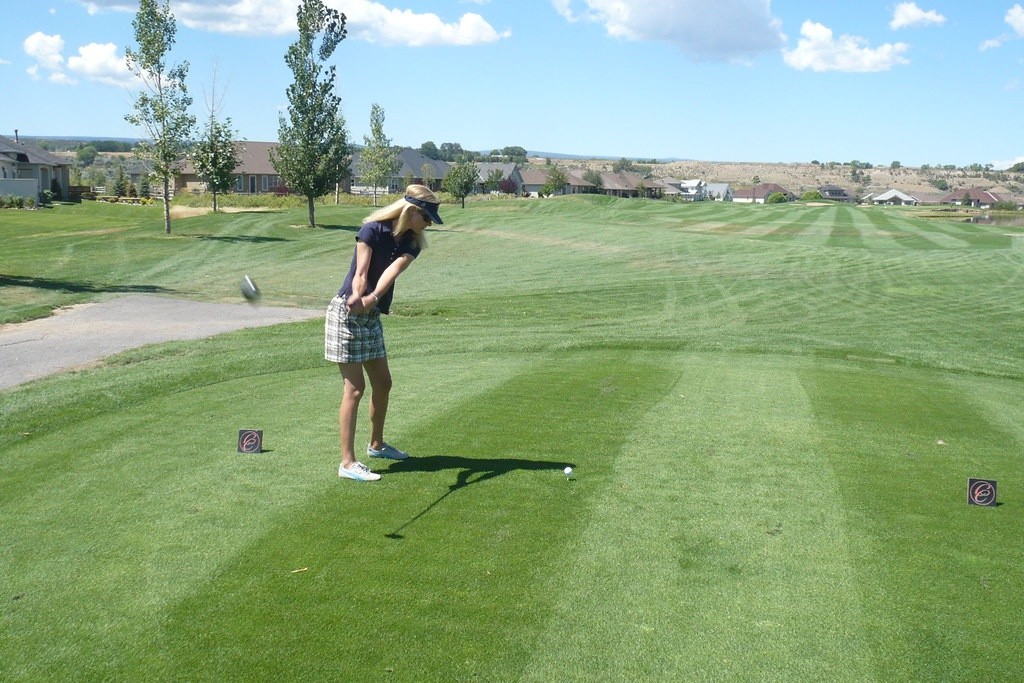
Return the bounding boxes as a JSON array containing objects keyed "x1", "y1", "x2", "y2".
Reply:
[{"x1": 564, "y1": 466, "x2": 572, "y2": 474}]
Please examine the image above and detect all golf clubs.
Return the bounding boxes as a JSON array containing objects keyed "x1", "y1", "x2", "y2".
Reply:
[{"x1": 239, "y1": 273, "x2": 357, "y2": 310}]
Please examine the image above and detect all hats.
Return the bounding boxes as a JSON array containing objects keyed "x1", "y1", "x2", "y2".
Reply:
[{"x1": 404, "y1": 195, "x2": 444, "y2": 225}]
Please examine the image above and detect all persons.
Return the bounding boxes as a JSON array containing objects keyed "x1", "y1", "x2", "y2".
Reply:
[{"x1": 324, "y1": 184, "x2": 444, "y2": 481}]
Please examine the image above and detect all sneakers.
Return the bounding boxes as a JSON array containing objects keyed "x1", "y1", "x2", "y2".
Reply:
[
  {"x1": 368, "y1": 441, "x2": 408, "y2": 460},
  {"x1": 338, "y1": 461, "x2": 382, "y2": 482}
]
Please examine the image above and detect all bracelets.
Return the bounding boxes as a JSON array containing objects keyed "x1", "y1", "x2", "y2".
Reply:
[{"x1": 371, "y1": 293, "x2": 379, "y2": 304}]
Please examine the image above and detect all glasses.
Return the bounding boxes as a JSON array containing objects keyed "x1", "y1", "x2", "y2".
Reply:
[{"x1": 419, "y1": 209, "x2": 431, "y2": 223}]
